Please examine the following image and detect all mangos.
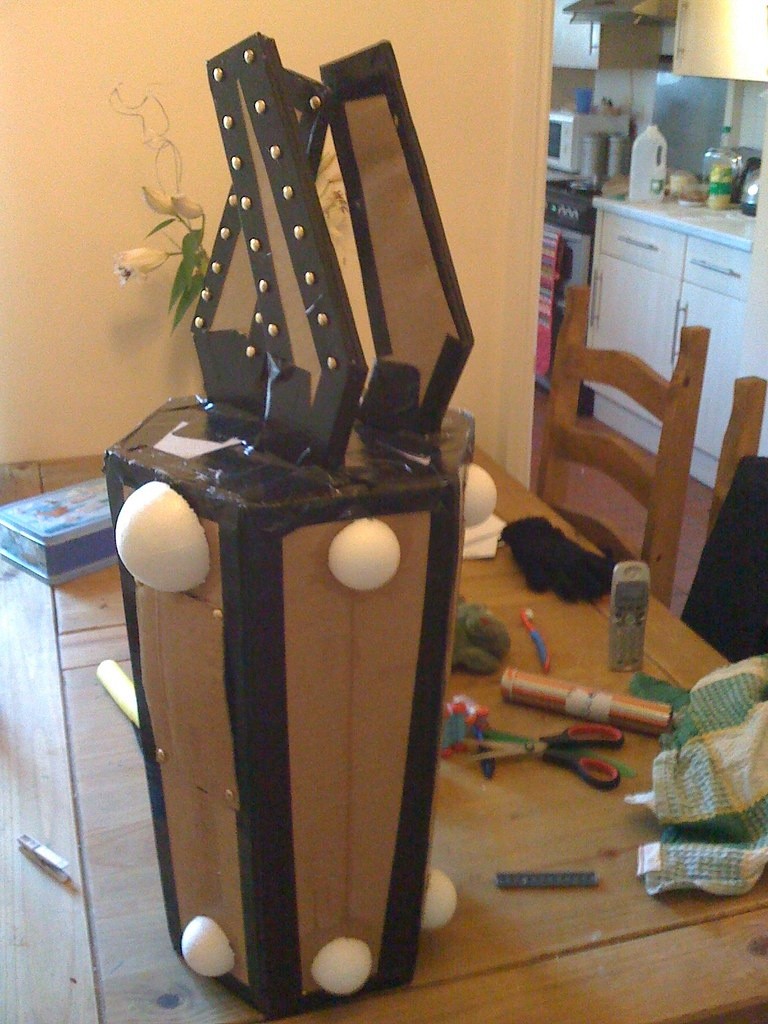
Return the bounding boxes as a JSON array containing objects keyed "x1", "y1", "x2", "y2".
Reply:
[{"x1": 451, "y1": 602, "x2": 509, "y2": 672}]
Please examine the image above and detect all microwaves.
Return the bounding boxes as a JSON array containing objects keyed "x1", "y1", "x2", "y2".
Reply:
[{"x1": 548, "y1": 111, "x2": 630, "y2": 172}]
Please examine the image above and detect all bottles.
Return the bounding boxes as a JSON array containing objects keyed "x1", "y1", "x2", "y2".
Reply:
[{"x1": 628, "y1": 123, "x2": 668, "y2": 204}]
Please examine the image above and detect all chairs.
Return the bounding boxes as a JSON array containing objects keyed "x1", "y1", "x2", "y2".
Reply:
[
  {"x1": 688, "y1": 377, "x2": 767, "y2": 662},
  {"x1": 535, "y1": 287, "x2": 712, "y2": 608}
]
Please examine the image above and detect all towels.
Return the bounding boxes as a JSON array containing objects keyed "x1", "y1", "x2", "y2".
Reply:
[{"x1": 621, "y1": 650, "x2": 768, "y2": 899}]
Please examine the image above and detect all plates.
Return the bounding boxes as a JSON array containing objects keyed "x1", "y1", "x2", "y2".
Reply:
[{"x1": 677, "y1": 198, "x2": 703, "y2": 206}]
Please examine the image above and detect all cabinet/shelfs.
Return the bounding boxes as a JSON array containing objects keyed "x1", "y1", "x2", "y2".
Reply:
[
  {"x1": 581, "y1": 214, "x2": 768, "y2": 485},
  {"x1": 552, "y1": 0, "x2": 768, "y2": 84}
]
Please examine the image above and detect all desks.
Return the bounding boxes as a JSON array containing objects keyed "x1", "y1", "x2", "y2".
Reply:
[{"x1": 0, "y1": 454, "x2": 768, "y2": 1024}]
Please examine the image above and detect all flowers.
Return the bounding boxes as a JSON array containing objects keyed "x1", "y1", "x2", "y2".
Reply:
[{"x1": 114, "y1": 191, "x2": 212, "y2": 332}]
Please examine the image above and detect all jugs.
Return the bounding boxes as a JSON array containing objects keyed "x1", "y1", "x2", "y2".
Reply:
[{"x1": 737, "y1": 155, "x2": 763, "y2": 215}]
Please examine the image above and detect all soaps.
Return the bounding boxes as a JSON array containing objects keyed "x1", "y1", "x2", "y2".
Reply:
[{"x1": 679, "y1": 190, "x2": 707, "y2": 202}]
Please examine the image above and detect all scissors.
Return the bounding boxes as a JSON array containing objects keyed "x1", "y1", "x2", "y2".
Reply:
[{"x1": 462, "y1": 723, "x2": 626, "y2": 791}]
